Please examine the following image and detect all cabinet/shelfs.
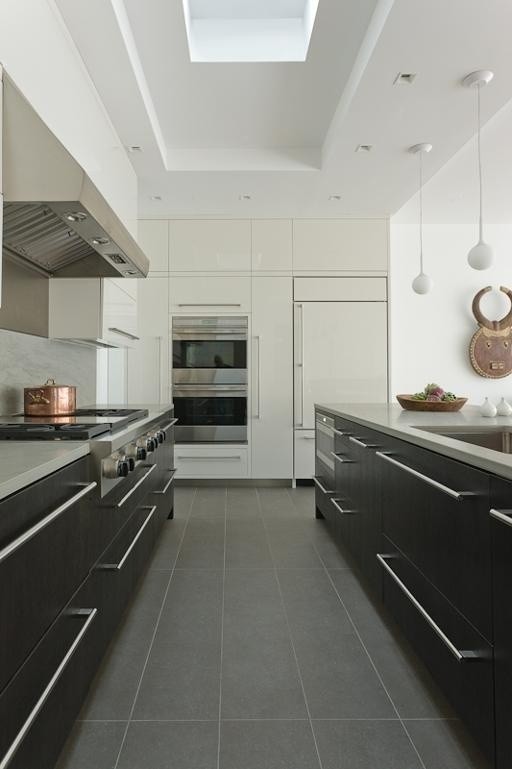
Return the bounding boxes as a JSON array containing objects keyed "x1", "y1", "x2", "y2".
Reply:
[
  {"x1": 173, "y1": 445, "x2": 248, "y2": 478},
  {"x1": 252, "y1": 277, "x2": 294, "y2": 480},
  {"x1": 48, "y1": 277, "x2": 140, "y2": 350},
  {"x1": 90, "y1": 409, "x2": 177, "y2": 660},
  {"x1": 0, "y1": 452, "x2": 101, "y2": 769},
  {"x1": 169, "y1": 276, "x2": 251, "y2": 312},
  {"x1": 127, "y1": 278, "x2": 169, "y2": 404},
  {"x1": 293, "y1": 276, "x2": 387, "y2": 487}
]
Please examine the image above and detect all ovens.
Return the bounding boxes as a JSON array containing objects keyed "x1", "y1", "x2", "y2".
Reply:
[{"x1": 172, "y1": 316, "x2": 250, "y2": 444}]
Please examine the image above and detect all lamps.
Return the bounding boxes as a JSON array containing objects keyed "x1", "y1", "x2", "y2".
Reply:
[
  {"x1": 408, "y1": 143, "x2": 434, "y2": 295},
  {"x1": 462, "y1": 70, "x2": 495, "y2": 270}
]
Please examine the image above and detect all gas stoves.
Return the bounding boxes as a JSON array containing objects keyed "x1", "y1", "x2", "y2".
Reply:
[{"x1": 0, "y1": 405, "x2": 168, "y2": 498}]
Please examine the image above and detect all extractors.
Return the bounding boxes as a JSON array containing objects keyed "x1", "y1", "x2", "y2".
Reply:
[{"x1": 1, "y1": 63, "x2": 151, "y2": 280}]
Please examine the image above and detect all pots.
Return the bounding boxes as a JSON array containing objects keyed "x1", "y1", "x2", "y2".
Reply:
[{"x1": 22, "y1": 376, "x2": 78, "y2": 416}]
